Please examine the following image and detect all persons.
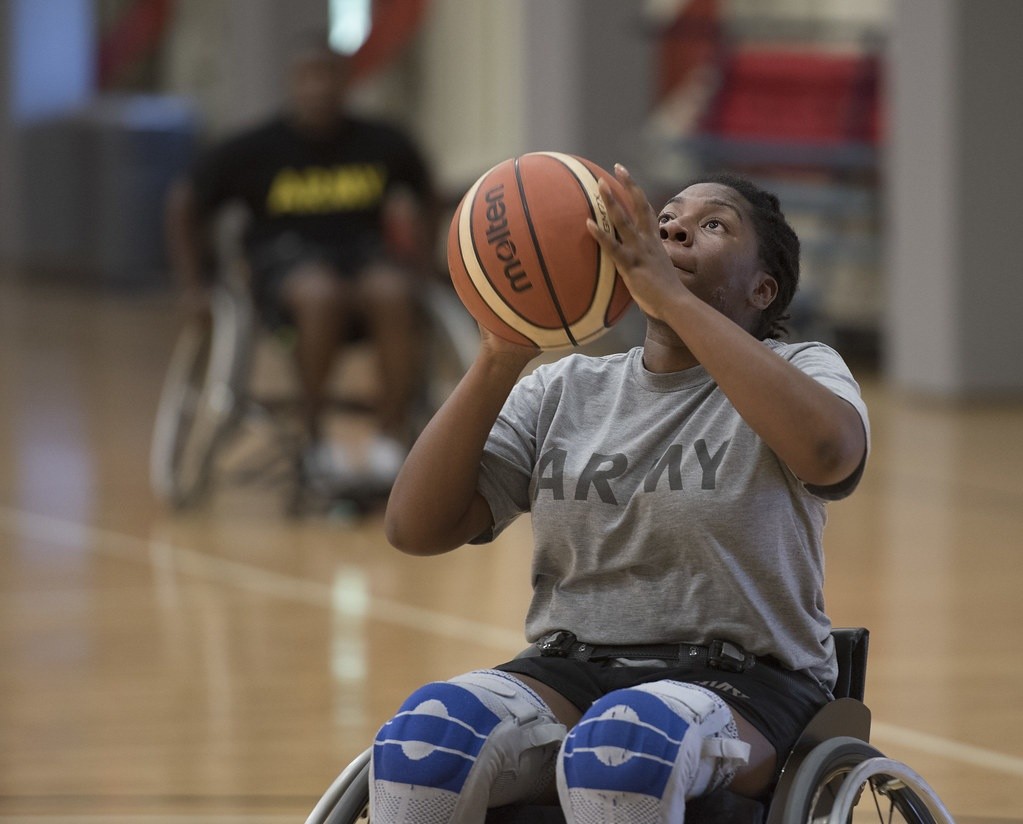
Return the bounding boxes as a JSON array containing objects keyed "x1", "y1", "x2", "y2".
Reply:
[
  {"x1": 372, "y1": 164, "x2": 869, "y2": 824},
  {"x1": 171, "y1": 56, "x2": 440, "y2": 516}
]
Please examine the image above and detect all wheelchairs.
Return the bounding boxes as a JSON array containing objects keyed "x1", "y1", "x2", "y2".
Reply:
[
  {"x1": 302, "y1": 620, "x2": 958, "y2": 824},
  {"x1": 144, "y1": 241, "x2": 479, "y2": 511}
]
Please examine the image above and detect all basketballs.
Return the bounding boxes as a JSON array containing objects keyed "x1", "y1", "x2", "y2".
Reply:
[{"x1": 445, "y1": 150, "x2": 635, "y2": 352}]
